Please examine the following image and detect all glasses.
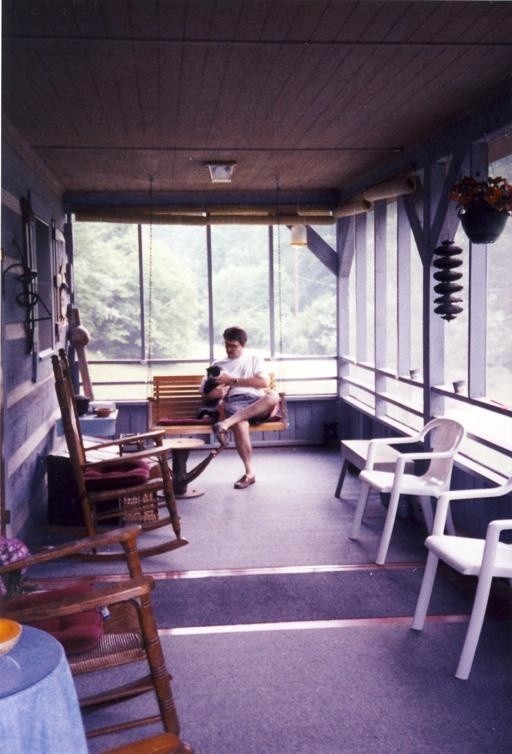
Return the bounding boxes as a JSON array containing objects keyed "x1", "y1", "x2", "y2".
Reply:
[{"x1": 222, "y1": 341, "x2": 243, "y2": 349}]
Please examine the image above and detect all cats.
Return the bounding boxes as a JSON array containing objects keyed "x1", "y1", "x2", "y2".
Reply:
[{"x1": 194, "y1": 365, "x2": 237, "y2": 424}]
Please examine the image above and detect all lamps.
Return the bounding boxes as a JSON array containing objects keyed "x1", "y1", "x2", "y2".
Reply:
[
  {"x1": 289, "y1": 196, "x2": 309, "y2": 247},
  {"x1": 205, "y1": 160, "x2": 238, "y2": 184}
]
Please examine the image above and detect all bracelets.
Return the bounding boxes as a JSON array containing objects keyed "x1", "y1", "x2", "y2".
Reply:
[{"x1": 233, "y1": 376, "x2": 240, "y2": 386}]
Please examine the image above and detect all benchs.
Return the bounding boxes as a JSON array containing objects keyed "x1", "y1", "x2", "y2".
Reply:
[{"x1": 145, "y1": 372, "x2": 291, "y2": 438}]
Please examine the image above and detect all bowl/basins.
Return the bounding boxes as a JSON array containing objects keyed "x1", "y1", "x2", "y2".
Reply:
[
  {"x1": 94, "y1": 407, "x2": 113, "y2": 417},
  {"x1": 0, "y1": 617, "x2": 23, "y2": 659}
]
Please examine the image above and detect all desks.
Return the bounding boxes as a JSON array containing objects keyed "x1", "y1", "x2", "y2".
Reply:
[{"x1": 1, "y1": 624, "x2": 89, "y2": 752}]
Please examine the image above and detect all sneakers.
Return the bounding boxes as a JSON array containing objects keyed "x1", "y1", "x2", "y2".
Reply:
[
  {"x1": 234, "y1": 475, "x2": 256, "y2": 489},
  {"x1": 213, "y1": 422, "x2": 231, "y2": 449}
]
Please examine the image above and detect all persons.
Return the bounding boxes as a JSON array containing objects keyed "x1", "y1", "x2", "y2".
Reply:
[{"x1": 197, "y1": 326, "x2": 282, "y2": 488}]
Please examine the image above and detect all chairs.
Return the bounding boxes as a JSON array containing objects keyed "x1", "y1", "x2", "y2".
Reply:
[
  {"x1": 105, "y1": 733, "x2": 191, "y2": 753},
  {"x1": 2, "y1": 459, "x2": 193, "y2": 737},
  {"x1": 403, "y1": 478, "x2": 510, "y2": 681},
  {"x1": 349, "y1": 417, "x2": 465, "y2": 565},
  {"x1": 51, "y1": 348, "x2": 191, "y2": 559}
]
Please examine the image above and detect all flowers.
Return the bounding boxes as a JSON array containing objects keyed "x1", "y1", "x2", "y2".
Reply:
[{"x1": 445, "y1": 174, "x2": 512, "y2": 217}]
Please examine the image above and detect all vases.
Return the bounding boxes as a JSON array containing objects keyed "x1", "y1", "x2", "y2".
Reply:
[{"x1": 456, "y1": 212, "x2": 510, "y2": 245}]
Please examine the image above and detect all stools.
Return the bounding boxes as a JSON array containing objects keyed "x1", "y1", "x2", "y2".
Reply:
[{"x1": 333, "y1": 437, "x2": 421, "y2": 526}]
